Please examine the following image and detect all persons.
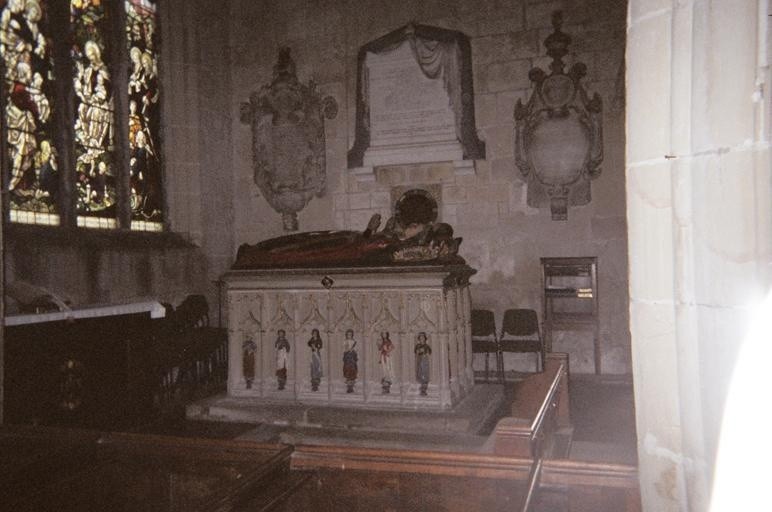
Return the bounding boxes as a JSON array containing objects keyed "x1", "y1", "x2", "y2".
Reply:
[
  {"x1": 376, "y1": 327, "x2": 394, "y2": 395},
  {"x1": 340, "y1": 326, "x2": 360, "y2": 394},
  {"x1": 305, "y1": 328, "x2": 323, "y2": 392},
  {"x1": 233, "y1": 212, "x2": 456, "y2": 268},
  {"x1": 0, "y1": 0, "x2": 163, "y2": 221},
  {"x1": 414, "y1": 330, "x2": 432, "y2": 397},
  {"x1": 242, "y1": 331, "x2": 259, "y2": 388},
  {"x1": 273, "y1": 329, "x2": 291, "y2": 389}
]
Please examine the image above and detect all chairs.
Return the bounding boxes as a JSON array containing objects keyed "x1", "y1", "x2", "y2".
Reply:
[
  {"x1": 153, "y1": 294, "x2": 226, "y2": 395},
  {"x1": 471, "y1": 308, "x2": 544, "y2": 384}
]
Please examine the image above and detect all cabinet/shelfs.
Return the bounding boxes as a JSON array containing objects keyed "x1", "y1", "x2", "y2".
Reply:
[{"x1": 541, "y1": 256, "x2": 602, "y2": 374}]
